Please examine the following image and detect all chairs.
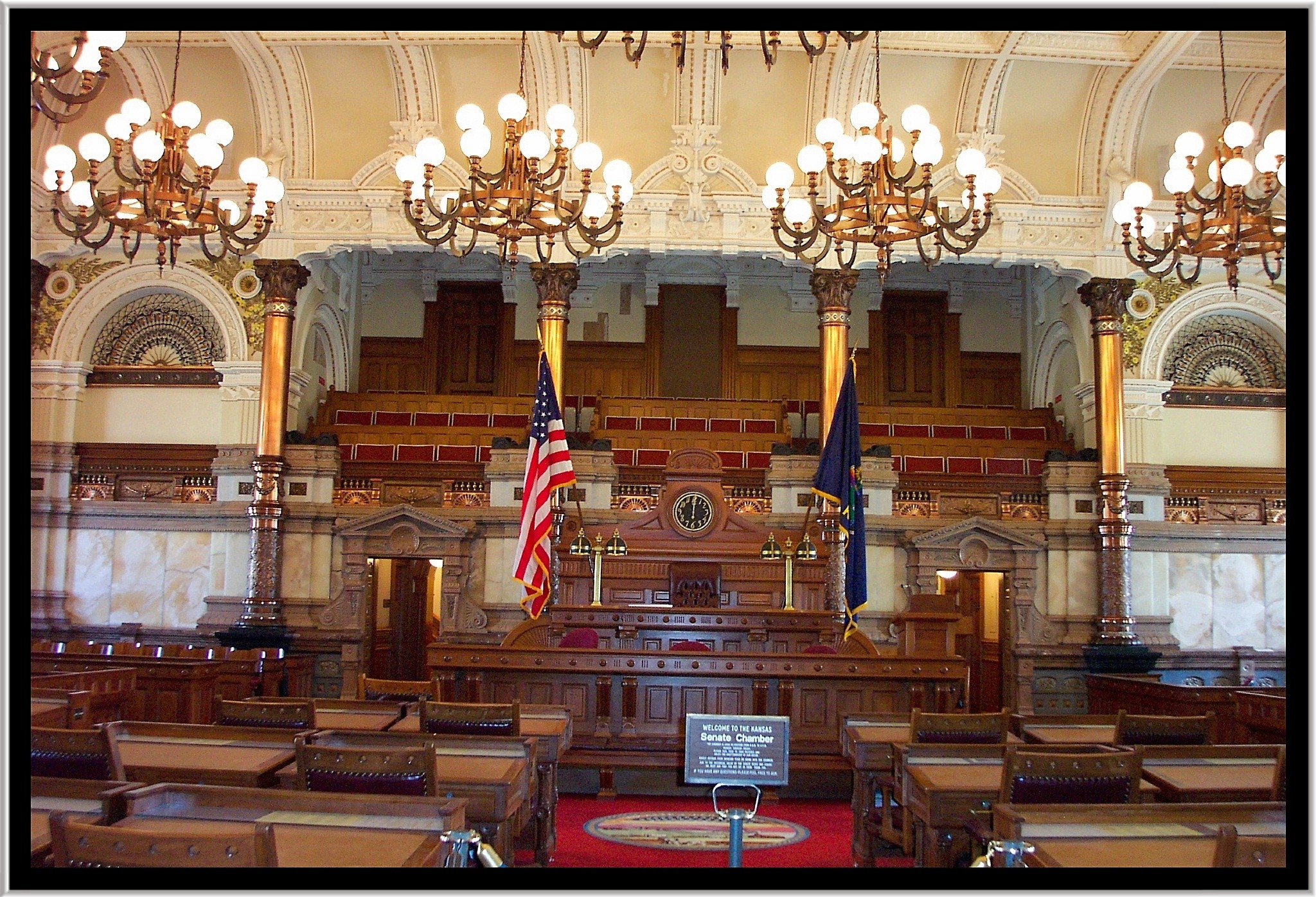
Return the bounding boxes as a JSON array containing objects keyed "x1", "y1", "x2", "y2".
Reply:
[
  {"x1": 1114, "y1": 709, "x2": 1215, "y2": 745},
  {"x1": 1213, "y1": 824, "x2": 1286, "y2": 867},
  {"x1": 668, "y1": 561, "x2": 721, "y2": 608},
  {"x1": 418, "y1": 697, "x2": 521, "y2": 737},
  {"x1": 669, "y1": 640, "x2": 711, "y2": 651},
  {"x1": 802, "y1": 645, "x2": 837, "y2": 655},
  {"x1": 355, "y1": 672, "x2": 442, "y2": 702},
  {"x1": 560, "y1": 627, "x2": 599, "y2": 649},
  {"x1": 294, "y1": 737, "x2": 439, "y2": 796},
  {"x1": 30, "y1": 722, "x2": 127, "y2": 782},
  {"x1": 864, "y1": 708, "x2": 1010, "y2": 867},
  {"x1": 49, "y1": 809, "x2": 280, "y2": 869},
  {"x1": 31, "y1": 641, "x2": 286, "y2": 660},
  {"x1": 964, "y1": 746, "x2": 1146, "y2": 868},
  {"x1": 214, "y1": 695, "x2": 315, "y2": 730}
]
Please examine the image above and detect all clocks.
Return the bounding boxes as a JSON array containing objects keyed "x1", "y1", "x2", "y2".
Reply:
[{"x1": 666, "y1": 485, "x2": 720, "y2": 539}]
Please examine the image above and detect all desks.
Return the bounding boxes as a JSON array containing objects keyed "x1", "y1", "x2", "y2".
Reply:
[
  {"x1": 30, "y1": 697, "x2": 573, "y2": 868},
  {"x1": 842, "y1": 723, "x2": 1285, "y2": 867},
  {"x1": 31, "y1": 652, "x2": 318, "y2": 729}
]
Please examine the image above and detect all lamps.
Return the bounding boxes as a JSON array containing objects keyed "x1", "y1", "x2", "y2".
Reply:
[
  {"x1": 761, "y1": 31, "x2": 1001, "y2": 286},
  {"x1": 544, "y1": 30, "x2": 870, "y2": 76},
  {"x1": 395, "y1": 30, "x2": 633, "y2": 275},
  {"x1": 1111, "y1": 31, "x2": 1286, "y2": 301},
  {"x1": 31, "y1": 30, "x2": 284, "y2": 278}
]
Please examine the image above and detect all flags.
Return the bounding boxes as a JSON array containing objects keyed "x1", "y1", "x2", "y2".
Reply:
[
  {"x1": 813, "y1": 358, "x2": 868, "y2": 640},
  {"x1": 514, "y1": 351, "x2": 578, "y2": 622}
]
